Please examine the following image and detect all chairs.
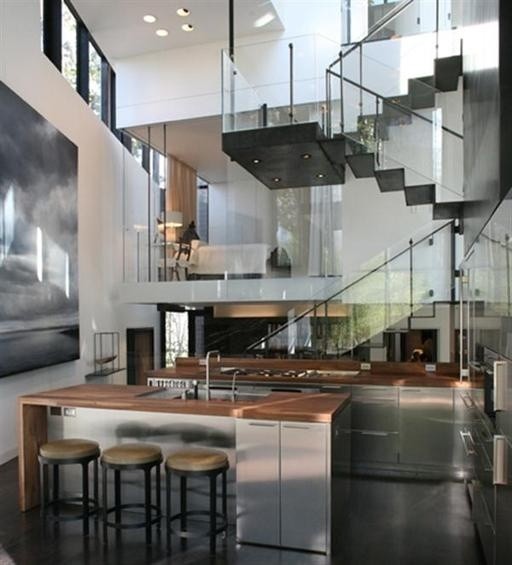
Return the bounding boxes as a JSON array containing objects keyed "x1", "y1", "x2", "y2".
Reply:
[{"x1": 159, "y1": 239, "x2": 191, "y2": 281}]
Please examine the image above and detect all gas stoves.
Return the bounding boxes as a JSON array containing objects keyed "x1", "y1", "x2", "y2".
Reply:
[{"x1": 220, "y1": 368, "x2": 306, "y2": 378}]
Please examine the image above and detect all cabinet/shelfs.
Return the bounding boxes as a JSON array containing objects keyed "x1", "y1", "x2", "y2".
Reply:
[
  {"x1": 323, "y1": 384, "x2": 454, "y2": 469},
  {"x1": 236, "y1": 420, "x2": 328, "y2": 553}
]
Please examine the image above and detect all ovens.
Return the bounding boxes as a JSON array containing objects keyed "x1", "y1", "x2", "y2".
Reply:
[{"x1": 458, "y1": 342, "x2": 512, "y2": 559}]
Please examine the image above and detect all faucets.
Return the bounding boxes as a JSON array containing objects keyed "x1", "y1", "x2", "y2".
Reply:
[
  {"x1": 229, "y1": 365, "x2": 248, "y2": 402},
  {"x1": 204, "y1": 347, "x2": 221, "y2": 402}
]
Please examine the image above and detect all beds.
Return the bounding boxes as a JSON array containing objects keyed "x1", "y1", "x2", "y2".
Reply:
[{"x1": 184, "y1": 221, "x2": 291, "y2": 278}]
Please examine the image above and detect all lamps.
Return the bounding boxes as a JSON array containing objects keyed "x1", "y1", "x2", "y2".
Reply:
[{"x1": 161, "y1": 211, "x2": 183, "y2": 242}]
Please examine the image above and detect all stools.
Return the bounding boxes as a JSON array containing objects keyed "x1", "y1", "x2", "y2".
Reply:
[{"x1": 35, "y1": 439, "x2": 229, "y2": 557}]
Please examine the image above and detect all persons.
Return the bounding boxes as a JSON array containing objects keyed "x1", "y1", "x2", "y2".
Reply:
[{"x1": 410, "y1": 338, "x2": 434, "y2": 362}]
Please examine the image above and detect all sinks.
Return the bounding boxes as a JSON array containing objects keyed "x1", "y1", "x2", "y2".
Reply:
[
  {"x1": 136, "y1": 385, "x2": 186, "y2": 402},
  {"x1": 185, "y1": 386, "x2": 241, "y2": 406}
]
